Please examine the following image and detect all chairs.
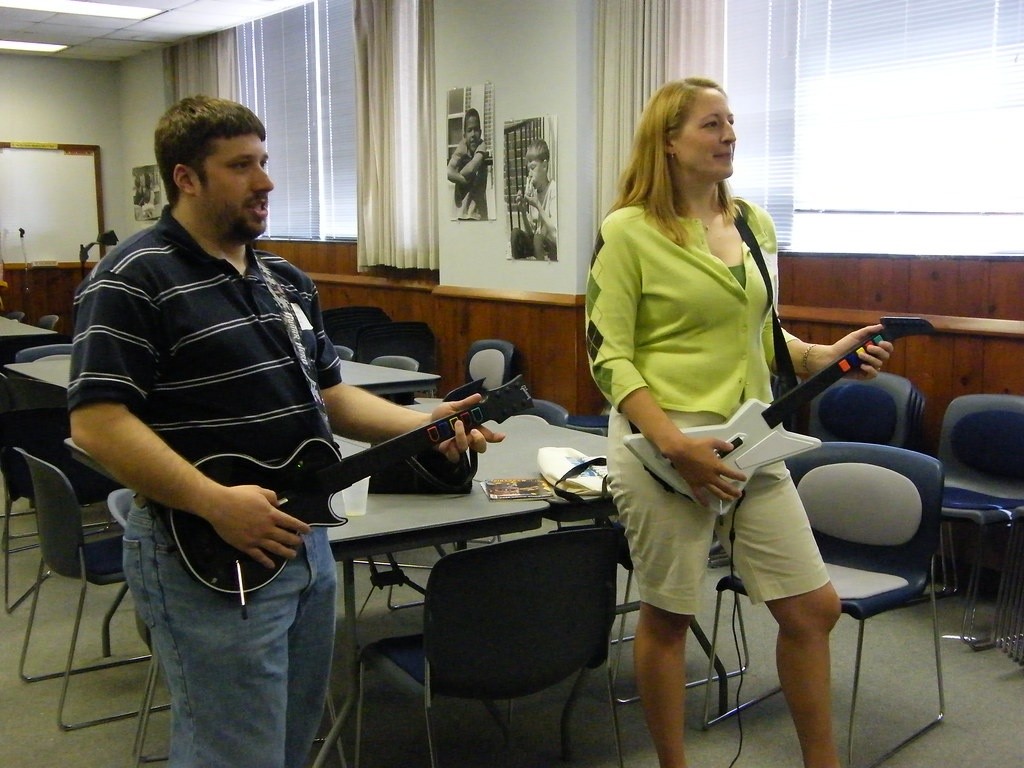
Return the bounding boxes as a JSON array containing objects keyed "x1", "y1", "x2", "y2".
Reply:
[
  {"x1": 1, "y1": 310, "x2": 751, "y2": 768},
  {"x1": 809, "y1": 371, "x2": 961, "y2": 610},
  {"x1": 702, "y1": 441, "x2": 947, "y2": 768},
  {"x1": 929, "y1": 393, "x2": 1024, "y2": 669}
]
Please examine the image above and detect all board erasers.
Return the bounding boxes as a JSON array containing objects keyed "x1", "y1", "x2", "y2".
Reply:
[{"x1": 31, "y1": 260, "x2": 60, "y2": 267}]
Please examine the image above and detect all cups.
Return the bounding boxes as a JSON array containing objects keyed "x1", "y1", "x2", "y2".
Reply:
[{"x1": 341, "y1": 476, "x2": 370, "y2": 518}]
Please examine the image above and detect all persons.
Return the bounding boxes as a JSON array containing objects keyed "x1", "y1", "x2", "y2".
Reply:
[
  {"x1": 584, "y1": 78, "x2": 893, "y2": 767},
  {"x1": 510, "y1": 139, "x2": 557, "y2": 260},
  {"x1": 67, "y1": 93, "x2": 506, "y2": 768},
  {"x1": 132, "y1": 172, "x2": 160, "y2": 206},
  {"x1": 445, "y1": 108, "x2": 489, "y2": 221}
]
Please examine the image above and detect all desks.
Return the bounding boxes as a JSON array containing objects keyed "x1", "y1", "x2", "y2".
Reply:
[
  {"x1": 5, "y1": 359, "x2": 69, "y2": 388},
  {"x1": 336, "y1": 358, "x2": 443, "y2": 397},
  {"x1": 402, "y1": 402, "x2": 729, "y2": 768},
  {"x1": 0, "y1": 316, "x2": 58, "y2": 338},
  {"x1": 312, "y1": 397, "x2": 549, "y2": 768}
]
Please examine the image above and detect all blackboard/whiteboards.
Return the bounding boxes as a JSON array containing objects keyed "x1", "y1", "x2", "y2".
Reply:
[{"x1": 0, "y1": 141, "x2": 107, "y2": 274}]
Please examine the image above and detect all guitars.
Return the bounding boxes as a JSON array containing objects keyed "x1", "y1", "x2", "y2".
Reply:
[
  {"x1": 624, "y1": 314, "x2": 937, "y2": 515},
  {"x1": 164, "y1": 373, "x2": 536, "y2": 596}
]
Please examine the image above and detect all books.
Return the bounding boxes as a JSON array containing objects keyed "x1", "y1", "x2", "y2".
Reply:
[{"x1": 481, "y1": 476, "x2": 554, "y2": 502}]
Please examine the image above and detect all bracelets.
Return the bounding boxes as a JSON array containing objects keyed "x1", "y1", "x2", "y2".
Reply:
[{"x1": 802, "y1": 344, "x2": 818, "y2": 373}]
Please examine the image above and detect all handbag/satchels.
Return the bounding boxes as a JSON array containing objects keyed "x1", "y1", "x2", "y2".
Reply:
[{"x1": 368, "y1": 443, "x2": 478, "y2": 494}]
